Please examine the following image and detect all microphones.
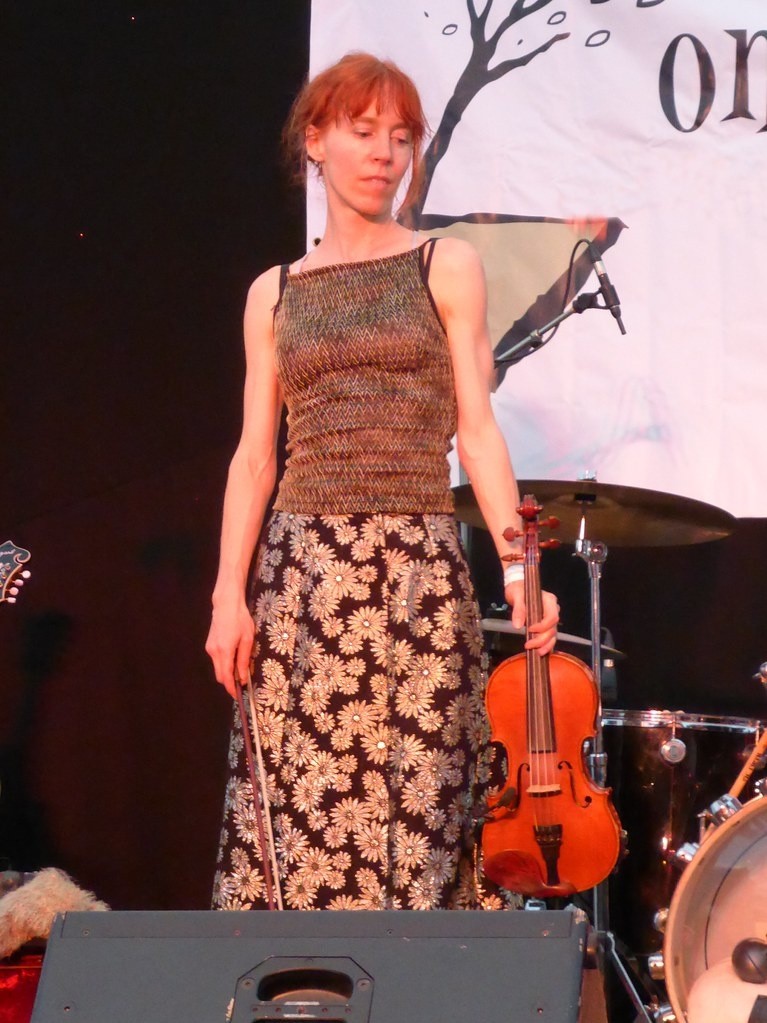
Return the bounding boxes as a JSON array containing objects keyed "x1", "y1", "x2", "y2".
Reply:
[
  {"x1": 733, "y1": 938, "x2": 767, "y2": 985},
  {"x1": 601, "y1": 633, "x2": 616, "y2": 701},
  {"x1": 588, "y1": 243, "x2": 626, "y2": 327}
]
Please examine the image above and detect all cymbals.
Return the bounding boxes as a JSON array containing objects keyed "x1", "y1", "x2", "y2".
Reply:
[
  {"x1": 451, "y1": 479, "x2": 741, "y2": 549},
  {"x1": 479, "y1": 615, "x2": 628, "y2": 663}
]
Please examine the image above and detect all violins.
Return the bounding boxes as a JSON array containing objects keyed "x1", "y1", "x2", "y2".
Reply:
[{"x1": 477, "y1": 492, "x2": 631, "y2": 899}]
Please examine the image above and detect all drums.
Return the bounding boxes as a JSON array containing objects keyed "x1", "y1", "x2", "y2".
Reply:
[
  {"x1": 662, "y1": 790, "x2": 767, "y2": 1023},
  {"x1": 596, "y1": 706, "x2": 765, "y2": 1022}
]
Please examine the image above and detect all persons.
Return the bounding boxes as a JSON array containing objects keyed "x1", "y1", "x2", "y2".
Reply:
[{"x1": 204, "y1": 54, "x2": 561, "y2": 914}]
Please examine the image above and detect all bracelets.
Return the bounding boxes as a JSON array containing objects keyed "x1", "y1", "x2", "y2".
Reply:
[{"x1": 503, "y1": 564, "x2": 525, "y2": 586}]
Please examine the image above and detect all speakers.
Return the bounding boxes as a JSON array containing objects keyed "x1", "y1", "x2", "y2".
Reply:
[{"x1": 30, "y1": 912, "x2": 588, "y2": 1022}]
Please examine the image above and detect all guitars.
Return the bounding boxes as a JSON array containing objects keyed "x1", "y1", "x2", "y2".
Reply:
[{"x1": 0, "y1": 538, "x2": 32, "y2": 605}]
